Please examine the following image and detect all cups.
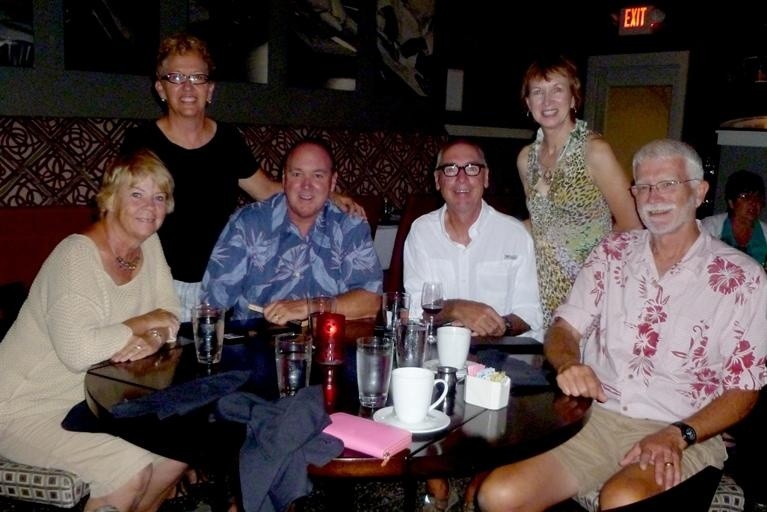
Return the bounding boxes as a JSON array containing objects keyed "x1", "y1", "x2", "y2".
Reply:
[
  {"x1": 355, "y1": 335, "x2": 394, "y2": 409},
  {"x1": 434, "y1": 326, "x2": 472, "y2": 371},
  {"x1": 274, "y1": 332, "x2": 312, "y2": 400},
  {"x1": 305, "y1": 292, "x2": 339, "y2": 337},
  {"x1": 421, "y1": 282, "x2": 445, "y2": 345},
  {"x1": 381, "y1": 291, "x2": 412, "y2": 337},
  {"x1": 190, "y1": 303, "x2": 225, "y2": 365},
  {"x1": 390, "y1": 367, "x2": 448, "y2": 424},
  {"x1": 395, "y1": 316, "x2": 429, "y2": 371}
]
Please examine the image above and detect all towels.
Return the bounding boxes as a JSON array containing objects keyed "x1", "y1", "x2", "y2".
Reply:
[
  {"x1": 110, "y1": 371, "x2": 251, "y2": 418},
  {"x1": 477, "y1": 349, "x2": 548, "y2": 386},
  {"x1": 216, "y1": 384, "x2": 344, "y2": 512}
]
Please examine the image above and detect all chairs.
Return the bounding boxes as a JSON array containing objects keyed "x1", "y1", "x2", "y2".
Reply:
[
  {"x1": 571, "y1": 475, "x2": 746, "y2": 510},
  {"x1": 0, "y1": 459, "x2": 89, "y2": 509}
]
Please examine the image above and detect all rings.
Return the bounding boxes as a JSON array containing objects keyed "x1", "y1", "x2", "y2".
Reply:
[{"x1": 136, "y1": 344, "x2": 144, "y2": 350}]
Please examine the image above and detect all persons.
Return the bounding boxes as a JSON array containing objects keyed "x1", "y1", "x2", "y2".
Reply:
[
  {"x1": 477, "y1": 138, "x2": 767, "y2": 512},
  {"x1": 403, "y1": 138, "x2": 544, "y2": 512},
  {"x1": 115, "y1": 33, "x2": 368, "y2": 282},
  {"x1": 196, "y1": 139, "x2": 383, "y2": 512},
  {"x1": 699, "y1": 168, "x2": 767, "y2": 274},
  {"x1": 0, "y1": 150, "x2": 193, "y2": 512},
  {"x1": 516, "y1": 56, "x2": 645, "y2": 344}
]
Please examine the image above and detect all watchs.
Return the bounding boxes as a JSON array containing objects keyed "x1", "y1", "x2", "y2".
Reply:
[
  {"x1": 671, "y1": 420, "x2": 697, "y2": 449},
  {"x1": 502, "y1": 316, "x2": 512, "y2": 335},
  {"x1": 150, "y1": 327, "x2": 165, "y2": 346}
]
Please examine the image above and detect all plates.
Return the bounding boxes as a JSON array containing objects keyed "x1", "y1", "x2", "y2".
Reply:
[
  {"x1": 424, "y1": 359, "x2": 485, "y2": 377},
  {"x1": 371, "y1": 405, "x2": 452, "y2": 435}
]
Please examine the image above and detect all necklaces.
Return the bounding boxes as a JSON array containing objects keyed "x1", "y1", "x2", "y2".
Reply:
[{"x1": 100, "y1": 215, "x2": 145, "y2": 274}]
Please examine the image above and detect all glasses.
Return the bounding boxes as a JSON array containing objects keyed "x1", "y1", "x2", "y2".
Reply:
[
  {"x1": 160, "y1": 72, "x2": 211, "y2": 87},
  {"x1": 437, "y1": 163, "x2": 487, "y2": 177},
  {"x1": 629, "y1": 178, "x2": 700, "y2": 197},
  {"x1": 739, "y1": 193, "x2": 763, "y2": 207}
]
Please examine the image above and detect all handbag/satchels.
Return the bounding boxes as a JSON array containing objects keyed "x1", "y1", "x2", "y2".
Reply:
[{"x1": 327, "y1": 412, "x2": 412, "y2": 466}]
